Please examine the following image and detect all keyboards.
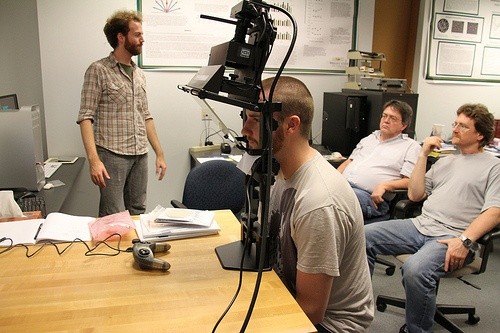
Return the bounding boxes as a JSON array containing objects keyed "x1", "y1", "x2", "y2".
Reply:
[
  {"x1": 43, "y1": 162, "x2": 62, "y2": 179},
  {"x1": 16, "y1": 197, "x2": 47, "y2": 218}
]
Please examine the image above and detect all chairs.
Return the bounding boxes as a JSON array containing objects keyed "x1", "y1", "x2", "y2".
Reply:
[
  {"x1": 375, "y1": 199, "x2": 500, "y2": 333},
  {"x1": 364, "y1": 155, "x2": 434, "y2": 276},
  {"x1": 170, "y1": 160, "x2": 247, "y2": 221}
]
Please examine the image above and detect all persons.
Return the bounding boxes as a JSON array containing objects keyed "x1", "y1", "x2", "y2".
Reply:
[
  {"x1": 337, "y1": 99, "x2": 425, "y2": 219},
  {"x1": 241, "y1": 76, "x2": 374, "y2": 333},
  {"x1": 364, "y1": 104, "x2": 500, "y2": 333},
  {"x1": 76, "y1": 11, "x2": 166, "y2": 218}
]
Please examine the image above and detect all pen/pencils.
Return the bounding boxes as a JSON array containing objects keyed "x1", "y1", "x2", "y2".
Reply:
[{"x1": 34, "y1": 223, "x2": 43, "y2": 239}]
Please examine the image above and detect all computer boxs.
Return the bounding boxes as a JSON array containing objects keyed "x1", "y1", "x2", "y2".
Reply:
[{"x1": 0, "y1": 104, "x2": 47, "y2": 193}]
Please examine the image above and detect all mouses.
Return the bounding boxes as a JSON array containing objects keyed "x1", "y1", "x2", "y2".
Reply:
[
  {"x1": 19, "y1": 192, "x2": 36, "y2": 198},
  {"x1": 43, "y1": 183, "x2": 54, "y2": 190}
]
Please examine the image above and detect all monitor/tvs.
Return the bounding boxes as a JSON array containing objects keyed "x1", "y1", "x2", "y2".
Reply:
[{"x1": 0, "y1": 93, "x2": 19, "y2": 111}]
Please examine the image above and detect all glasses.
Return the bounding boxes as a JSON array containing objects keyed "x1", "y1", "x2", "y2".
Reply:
[
  {"x1": 452, "y1": 121, "x2": 480, "y2": 134},
  {"x1": 379, "y1": 113, "x2": 403, "y2": 122}
]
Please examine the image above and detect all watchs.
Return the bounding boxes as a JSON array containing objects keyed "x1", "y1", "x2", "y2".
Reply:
[{"x1": 459, "y1": 235, "x2": 473, "y2": 249}]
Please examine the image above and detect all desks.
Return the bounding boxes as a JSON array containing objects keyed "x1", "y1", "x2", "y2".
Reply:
[
  {"x1": 189, "y1": 143, "x2": 349, "y2": 171},
  {"x1": 14, "y1": 156, "x2": 86, "y2": 218},
  {"x1": 0, "y1": 208, "x2": 319, "y2": 333}
]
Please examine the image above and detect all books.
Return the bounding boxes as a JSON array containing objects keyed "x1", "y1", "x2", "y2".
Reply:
[{"x1": 0, "y1": 205, "x2": 221, "y2": 247}]
